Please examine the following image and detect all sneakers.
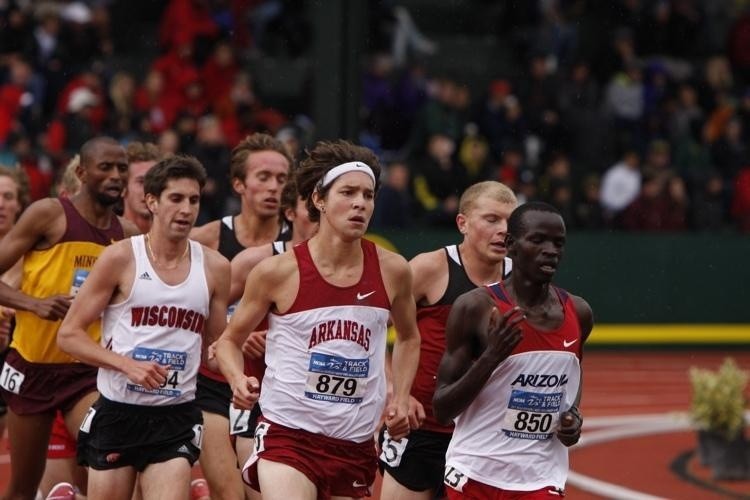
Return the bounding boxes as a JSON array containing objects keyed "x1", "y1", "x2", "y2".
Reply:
[
  {"x1": 46, "y1": 482, "x2": 75, "y2": 500},
  {"x1": 191, "y1": 478, "x2": 210, "y2": 500}
]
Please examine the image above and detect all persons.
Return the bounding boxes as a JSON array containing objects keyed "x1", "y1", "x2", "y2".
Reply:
[
  {"x1": 376, "y1": 180, "x2": 593, "y2": 500},
  {"x1": 0, "y1": 137, "x2": 230, "y2": 500},
  {"x1": 191, "y1": 132, "x2": 422, "y2": 500},
  {"x1": 0, "y1": 1, "x2": 750, "y2": 235}
]
